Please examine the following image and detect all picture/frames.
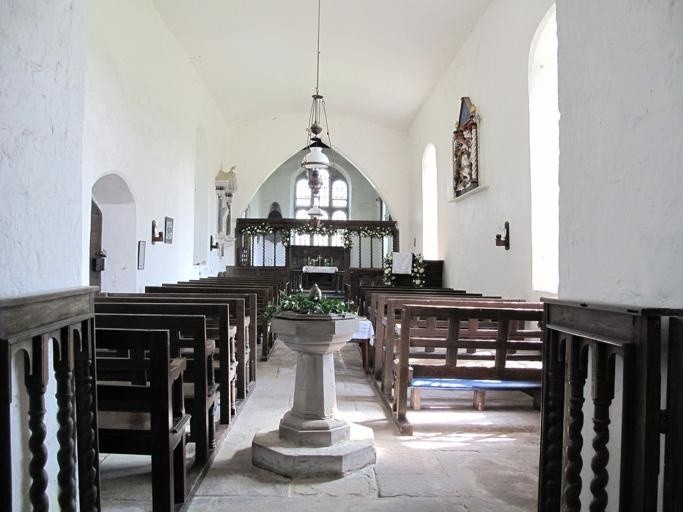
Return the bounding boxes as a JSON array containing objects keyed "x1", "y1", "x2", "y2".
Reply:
[
  {"x1": 164, "y1": 216, "x2": 174, "y2": 244},
  {"x1": 137, "y1": 241, "x2": 147, "y2": 270},
  {"x1": 450, "y1": 97, "x2": 486, "y2": 203}
]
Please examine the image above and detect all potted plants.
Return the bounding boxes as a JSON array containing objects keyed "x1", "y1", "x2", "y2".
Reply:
[{"x1": 249, "y1": 288, "x2": 379, "y2": 480}]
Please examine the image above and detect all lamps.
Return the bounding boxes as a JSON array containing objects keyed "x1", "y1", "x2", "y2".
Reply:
[{"x1": 302, "y1": 0, "x2": 332, "y2": 171}]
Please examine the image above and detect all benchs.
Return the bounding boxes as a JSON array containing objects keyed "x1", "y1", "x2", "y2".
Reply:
[
  {"x1": 143, "y1": 276, "x2": 296, "y2": 361},
  {"x1": 380, "y1": 299, "x2": 543, "y2": 406},
  {"x1": 354, "y1": 285, "x2": 482, "y2": 372},
  {"x1": 376, "y1": 295, "x2": 526, "y2": 394},
  {"x1": 95, "y1": 301, "x2": 239, "y2": 423},
  {"x1": 97, "y1": 312, "x2": 221, "y2": 464},
  {"x1": 96, "y1": 327, "x2": 191, "y2": 512},
  {"x1": 392, "y1": 303, "x2": 544, "y2": 435}
]
